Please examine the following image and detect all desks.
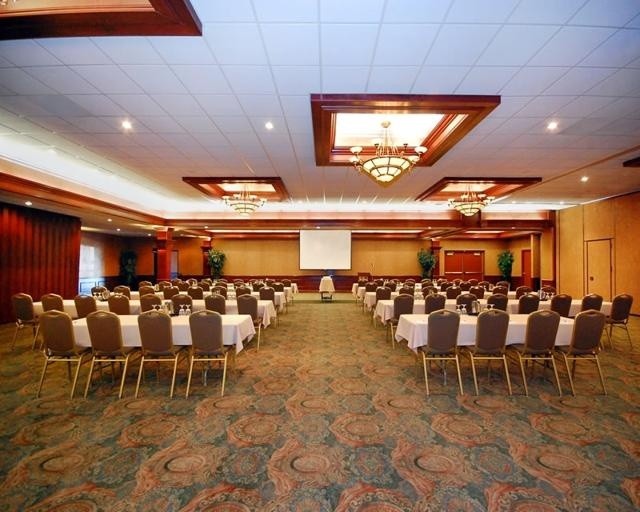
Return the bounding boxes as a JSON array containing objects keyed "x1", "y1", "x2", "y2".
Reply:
[{"x1": 320, "y1": 276, "x2": 335, "y2": 302}]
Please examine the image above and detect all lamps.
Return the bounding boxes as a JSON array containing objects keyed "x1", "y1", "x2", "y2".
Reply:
[
  {"x1": 350, "y1": 120, "x2": 428, "y2": 188},
  {"x1": 448, "y1": 183, "x2": 495, "y2": 216},
  {"x1": 222, "y1": 185, "x2": 266, "y2": 216}
]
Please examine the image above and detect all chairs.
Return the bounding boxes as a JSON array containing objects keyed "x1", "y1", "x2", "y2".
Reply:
[
  {"x1": 359, "y1": 277, "x2": 633, "y2": 398},
  {"x1": 10, "y1": 278, "x2": 290, "y2": 400}
]
